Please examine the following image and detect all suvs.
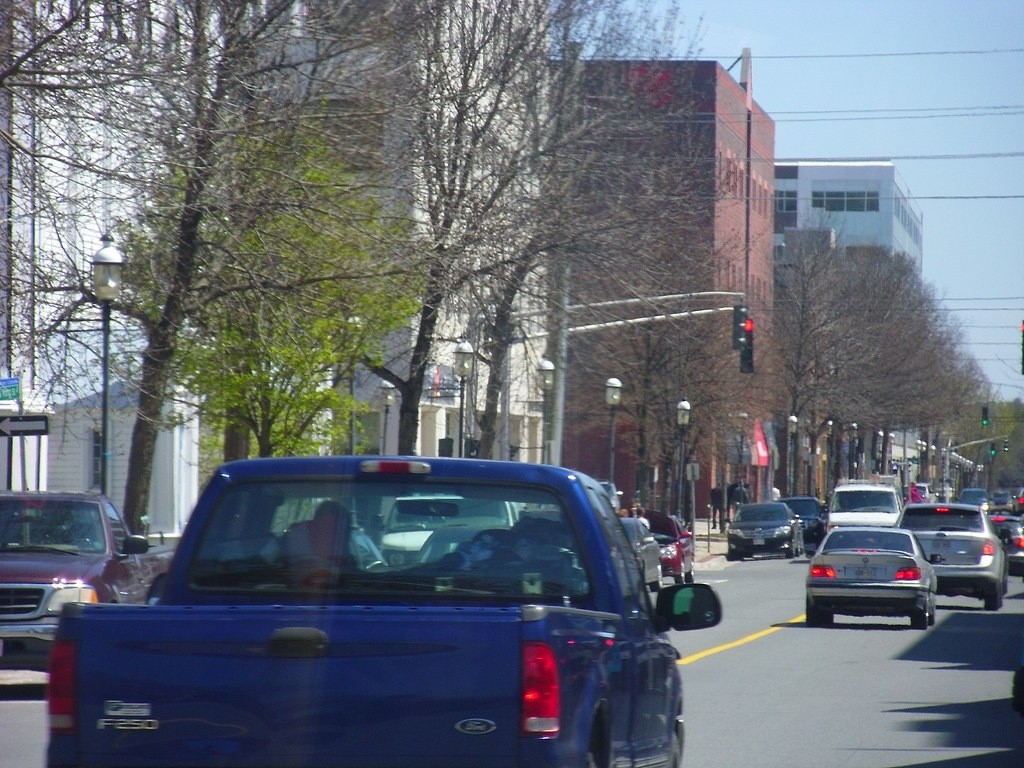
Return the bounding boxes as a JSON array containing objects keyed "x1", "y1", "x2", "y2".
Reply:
[
  {"x1": 903, "y1": 482, "x2": 938, "y2": 502},
  {"x1": 893, "y1": 502, "x2": 1011, "y2": 610}
]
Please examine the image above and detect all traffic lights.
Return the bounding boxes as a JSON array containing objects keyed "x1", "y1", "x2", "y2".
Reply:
[
  {"x1": 982, "y1": 407, "x2": 988, "y2": 425},
  {"x1": 739, "y1": 320, "x2": 754, "y2": 373},
  {"x1": 991, "y1": 443, "x2": 995, "y2": 456}
]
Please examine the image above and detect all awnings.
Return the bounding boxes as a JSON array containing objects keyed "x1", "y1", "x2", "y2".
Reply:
[{"x1": 722, "y1": 420, "x2": 779, "y2": 469}]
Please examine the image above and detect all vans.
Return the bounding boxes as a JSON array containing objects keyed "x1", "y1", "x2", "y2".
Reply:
[{"x1": 826, "y1": 478, "x2": 902, "y2": 534}]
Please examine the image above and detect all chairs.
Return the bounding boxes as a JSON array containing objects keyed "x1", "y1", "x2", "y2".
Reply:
[{"x1": 282, "y1": 522, "x2": 362, "y2": 575}]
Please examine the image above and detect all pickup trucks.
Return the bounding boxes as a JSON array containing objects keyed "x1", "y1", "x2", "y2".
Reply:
[
  {"x1": 0, "y1": 490, "x2": 181, "y2": 670},
  {"x1": 45, "y1": 453, "x2": 724, "y2": 768}
]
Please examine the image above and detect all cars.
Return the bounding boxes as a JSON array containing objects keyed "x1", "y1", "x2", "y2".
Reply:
[
  {"x1": 725, "y1": 496, "x2": 828, "y2": 561},
  {"x1": 806, "y1": 527, "x2": 944, "y2": 630},
  {"x1": 958, "y1": 487, "x2": 1024, "y2": 572},
  {"x1": 597, "y1": 481, "x2": 695, "y2": 592}
]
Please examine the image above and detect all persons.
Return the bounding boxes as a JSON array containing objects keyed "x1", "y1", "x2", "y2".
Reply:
[
  {"x1": 904, "y1": 481, "x2": 924, "y2": 504},
  {"x1": 710, "y1": 480, "x2": 751, "y2": 529},
  {"x1": 771, "y1": 484, "x2": 781, "y2": 500}
]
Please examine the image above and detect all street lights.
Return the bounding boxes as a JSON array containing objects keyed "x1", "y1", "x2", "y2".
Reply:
[
  {"x1": 738, "y1": 410, "x2": 833, "y2": 505},
  {"x1": 604, "y1": 377, "x2": 622, "y2": 482},
  {"x1": 849, "y1": 421, "x2": 983, "y2": 501},
  {"x1": 346, "y1": 314, "x2": 361, "y2": 453},
  {"x1": 378, "y1": 380, "x2": 396, "y2": 452},
  {"x1": 90, "y1": 235, "x2": 126, "y2": 495},
  {"x1": 452, "y1": 340, "x2": 474, "y2": 459},
  {"x1": 536, "y1": 360, "x2": 555, "y2": 464},
  {"x1": 677, "y1": 398, "x2": 690, "y2": 519}
]
